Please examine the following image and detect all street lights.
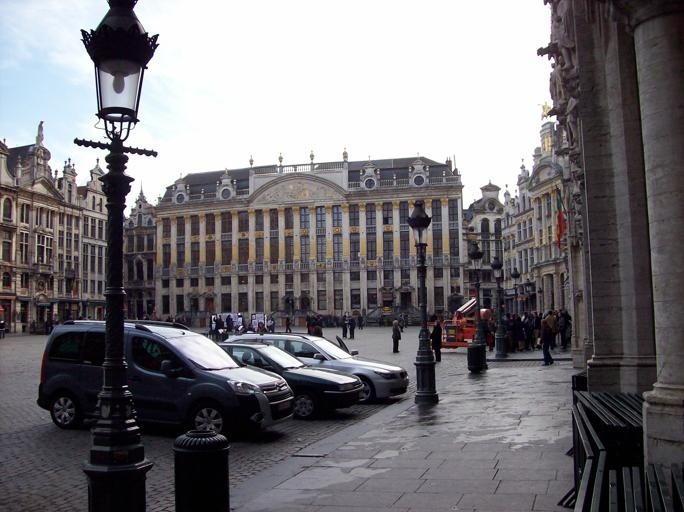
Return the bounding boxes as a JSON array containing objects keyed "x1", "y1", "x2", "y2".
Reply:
[
  {"x1": 80, "y1": 0, "x2": 159, "y2": 512},
  {"x1": 407, "y1": 200, "x2": 439, "y2": 403},
  {"x1": 468, "y1": 240, "x2": 520, "y2": 373}
]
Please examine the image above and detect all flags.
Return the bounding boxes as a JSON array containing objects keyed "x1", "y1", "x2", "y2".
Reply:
[{"x1": 552, "y1": 185, "x2": 566, "y2": 250}]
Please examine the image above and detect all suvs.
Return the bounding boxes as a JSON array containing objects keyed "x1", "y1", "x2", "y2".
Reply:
[{"x1": 37, "y1": 319, "x2": 294, "y2": 437}]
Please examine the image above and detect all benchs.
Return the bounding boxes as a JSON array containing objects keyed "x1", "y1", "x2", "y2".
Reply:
[{"x1": 556, "y1": 388, "x2": 684, "y2": 512}]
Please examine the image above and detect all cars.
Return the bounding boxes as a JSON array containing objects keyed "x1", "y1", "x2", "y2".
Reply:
[{"x1": 216, "y1": 332, "x2": 409, "y2": 418}]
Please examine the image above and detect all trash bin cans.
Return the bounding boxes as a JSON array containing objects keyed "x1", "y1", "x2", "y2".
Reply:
[
  {"x1": 170, "y1": 428, "x2": 232, "y2": 512},
  {"x1": 466, "y1": 343, "x2": 488, "y2": 374}
]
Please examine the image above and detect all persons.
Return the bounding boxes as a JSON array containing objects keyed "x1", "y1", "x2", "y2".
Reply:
[
  {"x1": 429, "y1": 319, "x2": 443, "y2": 362},
  {"x1": 538, "y1": 318, "x2": 554, "y2": 366},
  {"x1": 391, "y1": 320, "x2": 401, "y2": 353},
  {"x1": 551, "y1": 10, "x2": 584, "y2": 157},
  {"x1": 486, "y1": 306, "x2": 571, "y2": 354},
  {"x1": 0, "y1": 319, "x2": 5, "y2": 339},
  {"x1": 212, "y1": 310, "x2": 365, "y2": 341}
]
